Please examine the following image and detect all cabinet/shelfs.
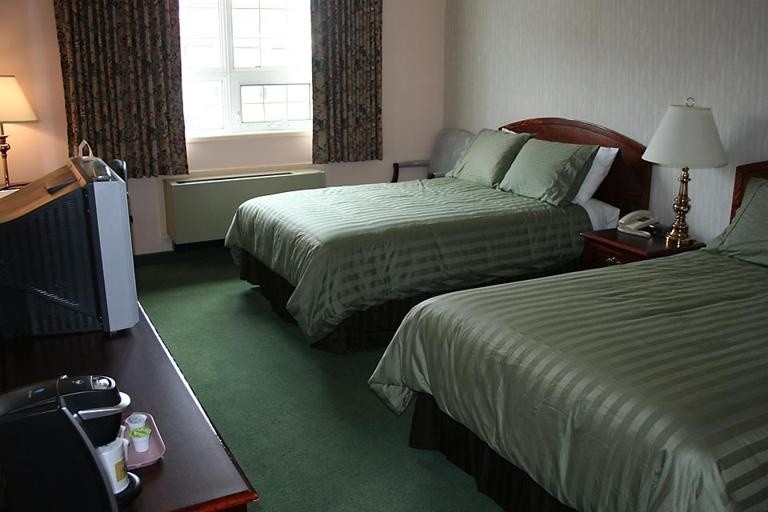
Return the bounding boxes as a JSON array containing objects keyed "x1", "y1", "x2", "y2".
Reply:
[
  {"x1": 161, "y1": 169, "x2": 327, "y2": 245},
  {"x1": 1, "y1": 303, "x2": 258, "y2": 512}
]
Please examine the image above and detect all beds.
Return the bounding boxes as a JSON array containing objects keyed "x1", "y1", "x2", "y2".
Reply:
[
  {"x1": 224, "y1": 119, "x2": 651, "y2": 345},
  {"x1": 367, "y1": 161, "x2": 768, "y2": 512}
]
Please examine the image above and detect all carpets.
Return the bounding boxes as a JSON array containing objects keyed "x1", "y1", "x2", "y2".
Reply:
[{"x1": 137, "y1": 252, "x2": 511, "y2": 512}]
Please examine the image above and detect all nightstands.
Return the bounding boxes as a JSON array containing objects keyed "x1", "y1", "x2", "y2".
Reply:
[{"x1": 579, "y1": 224, "x2": 705, "y2": 264}]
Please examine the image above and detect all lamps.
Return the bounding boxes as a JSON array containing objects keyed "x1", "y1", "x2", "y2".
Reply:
[
  {"x1": 641, "y1": 96, "x2": 728, "y2": 244},
  {"x1": 0, "y1": 76, "x2": 37, "y2": 191}
]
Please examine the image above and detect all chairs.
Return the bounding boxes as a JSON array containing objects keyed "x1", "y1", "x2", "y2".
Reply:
[{"x1": 391, "y1": 128, "x2": 476, "y2": 183}]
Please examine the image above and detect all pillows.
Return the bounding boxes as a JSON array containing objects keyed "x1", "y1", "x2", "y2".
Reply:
[
  {"x1": 499, "y1": 137, "x2": 619, "y2": 206},
  {"x1": 445, "y1": 128, "x2": 536, "y2": 189},
  {"x1": 571, "y1": 143, "x2": 618, "y2": 209},
  {"x1": 703, "y1": 177, "x2": 768, "y2": 267}
]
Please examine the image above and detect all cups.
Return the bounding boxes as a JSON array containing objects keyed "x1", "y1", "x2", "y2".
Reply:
[
  {"x1": 124, "y1": 414, "x2": 147, "y2": 431},
  {"x1": 96, "y1": 437, "x2": 130, "y2": 495},
  {"x1": 126, "y1": 427, "x2": 152, "y2": 452}
]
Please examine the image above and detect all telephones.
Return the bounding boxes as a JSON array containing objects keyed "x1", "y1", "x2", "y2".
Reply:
[{"x1": 618, "y1": 210, "x2": 659, "y2": 238}]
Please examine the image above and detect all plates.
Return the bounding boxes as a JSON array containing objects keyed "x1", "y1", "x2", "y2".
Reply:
[{"x1": 122, "y1": 412, "x2": 166, "y2": 470}]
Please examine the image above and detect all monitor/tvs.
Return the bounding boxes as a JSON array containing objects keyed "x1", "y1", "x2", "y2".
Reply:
[{"x1": 0, "y1": 156, "x2": 141, "y2": 336}]
